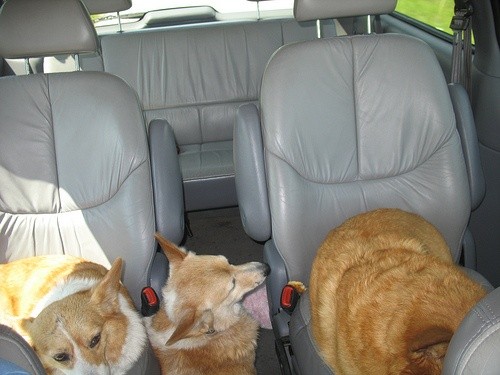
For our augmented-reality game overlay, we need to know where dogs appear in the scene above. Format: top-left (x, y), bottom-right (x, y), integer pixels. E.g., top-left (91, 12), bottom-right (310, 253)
top-left (308, 208), bottom-right (490, 375)
top-left (0, 254), bottom-right (149, 375)
top-left (140, 232), bottom-right (273, 375)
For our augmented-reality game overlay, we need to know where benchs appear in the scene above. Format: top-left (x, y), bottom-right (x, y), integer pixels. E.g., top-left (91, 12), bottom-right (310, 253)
top-left (8, 1), bottom-right (355, 212)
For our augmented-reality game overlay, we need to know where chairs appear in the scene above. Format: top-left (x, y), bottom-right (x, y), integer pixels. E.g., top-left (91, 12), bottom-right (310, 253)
top-left (0, 0), bottom-right (499, 375)
